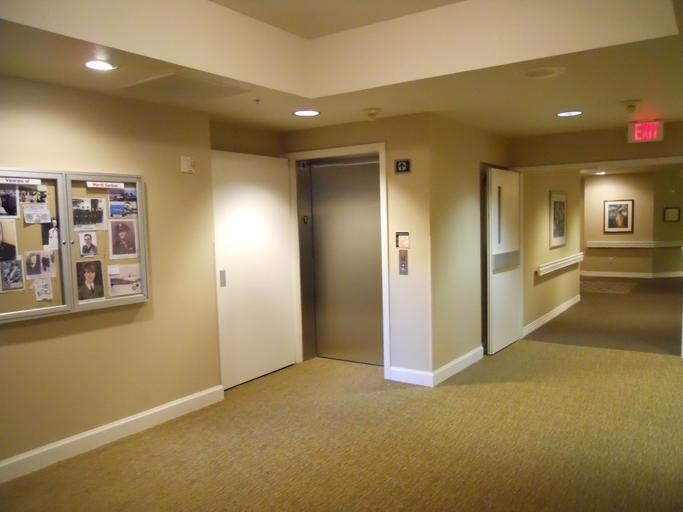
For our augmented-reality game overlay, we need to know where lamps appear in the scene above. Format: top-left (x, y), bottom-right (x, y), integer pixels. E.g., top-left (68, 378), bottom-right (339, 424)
top-left (363, 107), bottom-right (381, 119)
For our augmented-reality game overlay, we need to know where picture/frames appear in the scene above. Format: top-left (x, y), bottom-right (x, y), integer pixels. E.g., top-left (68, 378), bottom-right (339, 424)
top-left (549, 190), bottom-right (567, 249)
top-left (663, 207), bottom-right (680, 222)
top-left (603, 199), bottom-right (634, 233)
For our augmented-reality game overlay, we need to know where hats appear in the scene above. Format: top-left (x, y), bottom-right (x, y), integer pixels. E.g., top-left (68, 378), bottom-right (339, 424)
top-left (116, 222), bottom-right (129, 233)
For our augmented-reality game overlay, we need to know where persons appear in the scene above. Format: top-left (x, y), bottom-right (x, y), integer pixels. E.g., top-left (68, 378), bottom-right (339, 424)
top-left (81, 233), bottom-right (96, 254)
top-left (73, 204), bottom-right (103, 226)
top-left (26, 254), bottom-right (41, 277)
top-left (77, 261), bottom-right (102, 301)
top-left (17, 187), bottom-right (47, 204)
top-left (111, 221), bottom-right (135, 255)
top-left (5, 260), bottom-right (21, 286)
top-left (47, 219), bottom-right (59, 246)
